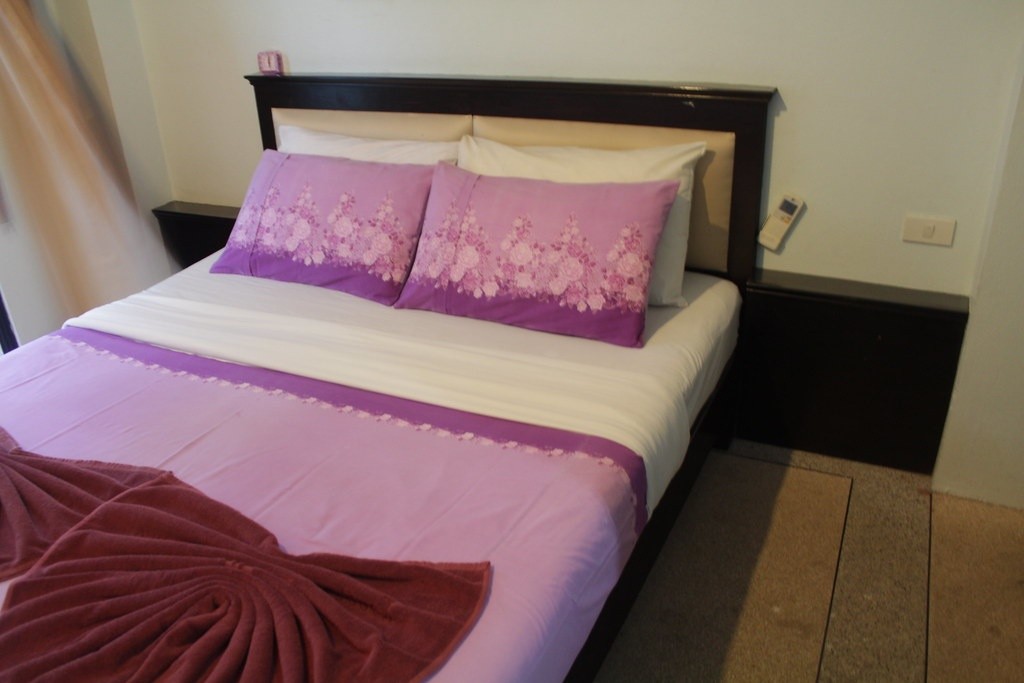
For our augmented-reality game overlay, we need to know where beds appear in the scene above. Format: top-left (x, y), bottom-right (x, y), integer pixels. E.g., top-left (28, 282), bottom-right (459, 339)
top-left (1, 74), bottom-right (778, 683)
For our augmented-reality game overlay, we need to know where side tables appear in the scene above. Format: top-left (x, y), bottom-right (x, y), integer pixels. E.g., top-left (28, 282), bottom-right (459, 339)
top-left (715, 268), bottom-right (970, 477)
top-left (150, 199), bottom-right (243, 267)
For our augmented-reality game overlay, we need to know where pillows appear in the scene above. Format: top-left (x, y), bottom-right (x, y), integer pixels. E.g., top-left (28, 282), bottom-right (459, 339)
top-left (211, 148), bottom-right (457, 308)
top-left (452, 135), bottom-right (707, 310)
top-left (394, 160), bottom-right (680, 351)
top-left (277, 122), bottom-right (457, 166)
